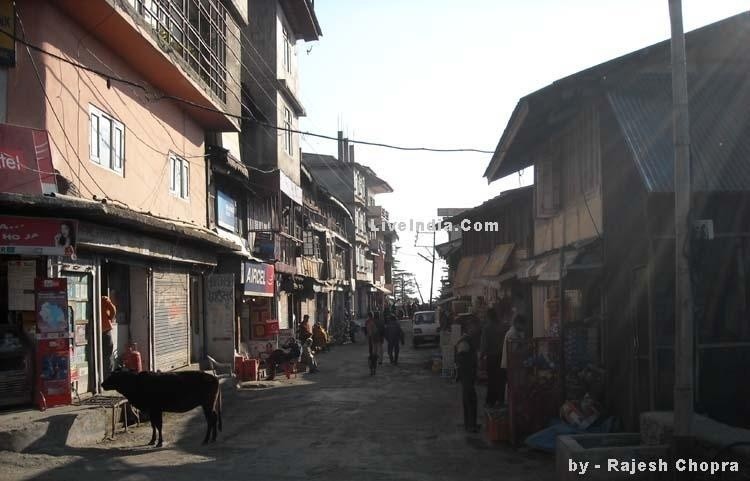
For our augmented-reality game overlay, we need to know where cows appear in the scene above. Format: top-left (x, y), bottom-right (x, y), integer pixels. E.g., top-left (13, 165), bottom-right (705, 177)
top-left (100, 364), bottom-right (223, 449)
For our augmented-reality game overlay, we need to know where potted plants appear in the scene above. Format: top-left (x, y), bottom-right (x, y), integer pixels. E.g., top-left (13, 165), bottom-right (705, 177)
top-left (487, 410), bottom-right (526, 441)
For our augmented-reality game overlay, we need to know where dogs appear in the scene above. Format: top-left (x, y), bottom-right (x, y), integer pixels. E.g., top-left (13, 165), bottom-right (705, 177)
top-left (368, 353), bottom-right (378, 375)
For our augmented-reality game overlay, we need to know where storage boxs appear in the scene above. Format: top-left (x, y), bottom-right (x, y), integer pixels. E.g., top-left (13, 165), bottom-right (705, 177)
top-left (429, 321), bottom-right (462, 377)
top-left (236, 357), bottom-right (272, 380)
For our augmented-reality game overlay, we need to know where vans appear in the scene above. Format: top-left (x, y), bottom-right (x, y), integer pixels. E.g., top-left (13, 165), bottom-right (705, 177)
top-left (411, 309), bottom-right (441, 344)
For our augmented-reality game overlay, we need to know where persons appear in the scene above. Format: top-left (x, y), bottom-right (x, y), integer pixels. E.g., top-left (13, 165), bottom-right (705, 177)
top-left (302, 315), bottom-right (311, 338)
top-left (367, 303), bottom-right (416, 378)
top-left (524, 358), bottom-right (606, 455)
top-left (453, 317), bottom-right (481, 434)
top-left (53, 222), bottom-right (75, 249)
top-left (478, 305), bottom-right (510, 410)
top-left (301, 338), bottom-right (320, 373)
top-left (257, 337), bottom-right (300, 380)
top-left (498, 313), bottom-right (527, 371)
